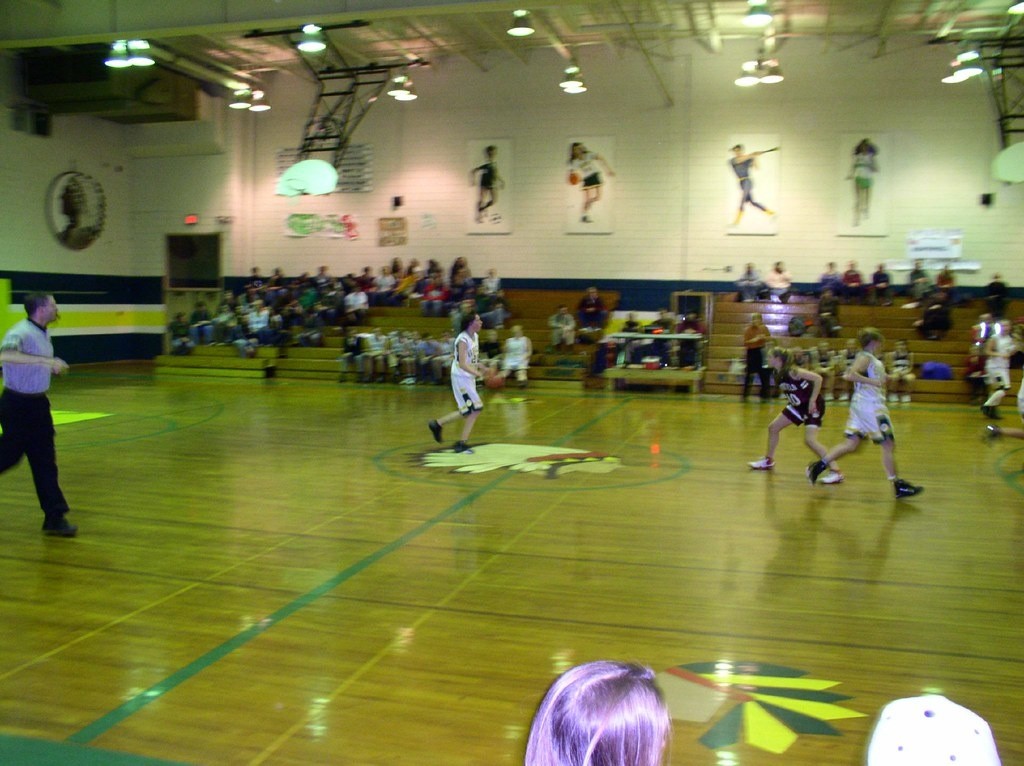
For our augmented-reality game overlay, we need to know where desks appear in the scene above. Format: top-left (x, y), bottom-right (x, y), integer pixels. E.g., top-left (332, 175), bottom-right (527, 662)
top-left (604, 330), bottom-right (706, 395)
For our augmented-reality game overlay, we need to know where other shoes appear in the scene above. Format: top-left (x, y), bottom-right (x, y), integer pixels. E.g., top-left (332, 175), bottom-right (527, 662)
top-left (454, 443), bottom-right (473, 454)
top-left (889, 393), bottom-right (899, 402)
top-left (901, 395), bottom-right (911, 403)
top-left (41, 515), bottom-right (78, 536)
top-left (428, 419), bottom-right (443, 445)
top-left (980, 405), bottom-right (1003, 418)
top-left (337, 371), bottom-right (451, 385)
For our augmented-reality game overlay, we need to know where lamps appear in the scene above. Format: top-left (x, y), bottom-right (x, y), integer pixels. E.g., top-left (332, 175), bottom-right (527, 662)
top-left (742, 0), bottom-right (771, 27)
top-left (734, 45), bottom-right (784, 86)
top-left (506, 9), bottom-right (536, 36)
top-left (559, 58), bottom-right (588, 94)
top-left (229, 88), bottom-right (272, 112)
top-left (298, 24), bottom-right (326, 53)
top-left (106, 39), bottom-right (155, 67)
top-left (387, 75), bottom-right (417, 101)
top-left (941, 41), bottom-right (983, 83)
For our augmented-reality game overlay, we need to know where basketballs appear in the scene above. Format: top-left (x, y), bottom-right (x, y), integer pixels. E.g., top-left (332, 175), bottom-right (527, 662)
top-left (484, 373), bottom-right (504, 389)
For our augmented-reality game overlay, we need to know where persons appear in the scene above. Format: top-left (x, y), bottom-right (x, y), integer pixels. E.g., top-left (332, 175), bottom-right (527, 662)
top-left (767, 341), bottom-right (916, 403)
top-left (819, 258), bottom-right (956, 308)
top-left (524, 660), bottom-right (674, 766)
top-left (729, 143), bottom-right (782, 226)
top-left (762, 261), bottom-right (793, 303)
top-left (738, 263), bottom-right (760, 303)
top-left (982, 272), bottom-right (1012, 320)
top-left (867, 694), bottom-right (1001, 766)
top-left (914, 298), bottom-right (954, 340)
top-left (428, 309), bottom-right (495, 452)
top-left (818, 286), bottom-right (842, 338)
top-left (959, 314), bottom-right (1024, 423)
top-left (0, 291), bottom-right (79, 537)
top-left (806, 327), bottom-right (924, 499)
top-left (612, 307), bottom-right (706, 370)
top-left (846, 138), bottom-right (879, 226)
top-left (339, 325), bottom-right (533, 389)
top-left (567, 142), bottom-right (615, 224)
top-left (470, 145), bottom-right (505, 224)
top-left (577, 287), bottom-right (608, 332)
top-left (166, 256), bottom-right (512, 360)
top-left (547, 302), bottom-right (577, 355)
top-left (739, 314), bottom-right (773, 403)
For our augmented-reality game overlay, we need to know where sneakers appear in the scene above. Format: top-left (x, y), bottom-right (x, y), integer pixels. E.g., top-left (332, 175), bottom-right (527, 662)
top-left (805, 460), bottom-right (827, 485)
top-left (821, 472), bottom-right (845, 484)
top-left (747, 458), bottom-right (775, 470)
top-left (893, 479), bottom-right (922, 498)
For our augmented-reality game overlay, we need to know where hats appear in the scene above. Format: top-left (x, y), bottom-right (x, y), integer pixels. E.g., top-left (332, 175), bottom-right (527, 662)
top-left (868, 694), bottom-right (1001, 766)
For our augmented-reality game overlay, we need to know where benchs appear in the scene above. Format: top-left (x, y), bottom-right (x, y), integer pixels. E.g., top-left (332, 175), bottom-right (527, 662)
top-left (153, 290), bottom-right (621, 380)
top-left (702, 290), bottom-right (1024, 406)
